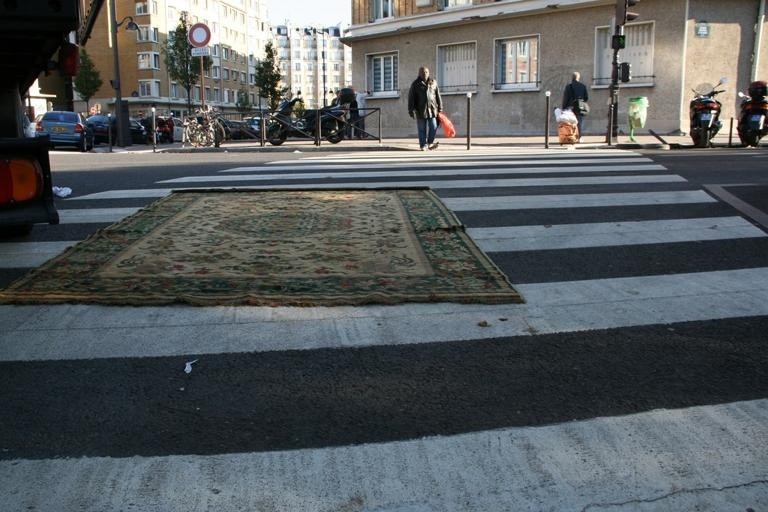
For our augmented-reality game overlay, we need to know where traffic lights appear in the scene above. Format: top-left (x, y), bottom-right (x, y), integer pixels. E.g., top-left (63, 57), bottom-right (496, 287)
top-left (611, 34), bottom-right (626, 50)
top-left (616, 1), bottom-right (639, 28)
top-left (622, 61), bottom-right (632, 84)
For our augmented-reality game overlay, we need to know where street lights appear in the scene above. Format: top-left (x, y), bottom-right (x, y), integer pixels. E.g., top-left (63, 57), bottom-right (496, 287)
top-left (107, 15), bottom-right (139, 146)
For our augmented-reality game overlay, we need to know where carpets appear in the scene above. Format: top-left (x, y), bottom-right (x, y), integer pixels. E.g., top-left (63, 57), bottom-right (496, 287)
top-left (1, 184), bottom-right (528, 307)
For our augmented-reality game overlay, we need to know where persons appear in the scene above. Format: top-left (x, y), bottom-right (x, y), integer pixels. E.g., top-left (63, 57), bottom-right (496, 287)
top-left (408, 67), bottom-right (442, 151)
top-left (347, 85), bottom-right (371, 135)
top-left (562, 72), bottom-right (588, 140)
top-left (331, 91), bottom-right (344, 136)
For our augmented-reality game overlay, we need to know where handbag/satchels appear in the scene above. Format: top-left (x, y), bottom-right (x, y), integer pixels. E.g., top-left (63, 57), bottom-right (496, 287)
top-left (572, 97), bottom-right (590, 117)
top-left (437, 110), bottom-right (456, 138)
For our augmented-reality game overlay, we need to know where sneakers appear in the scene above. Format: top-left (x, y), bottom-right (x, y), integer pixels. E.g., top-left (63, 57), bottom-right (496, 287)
top-left (420, 141), bottom-right (439, 152)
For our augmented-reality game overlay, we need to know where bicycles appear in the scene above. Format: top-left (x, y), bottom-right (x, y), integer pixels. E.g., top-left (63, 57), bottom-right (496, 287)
top-left (182, 105), bottom-right (227, 149)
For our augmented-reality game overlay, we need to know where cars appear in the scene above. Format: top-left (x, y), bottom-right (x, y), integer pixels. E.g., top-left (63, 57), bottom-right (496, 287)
top-left (27, 111), bottom-right (182, 152)
top-left (225, 114), bottom-right (306, 139)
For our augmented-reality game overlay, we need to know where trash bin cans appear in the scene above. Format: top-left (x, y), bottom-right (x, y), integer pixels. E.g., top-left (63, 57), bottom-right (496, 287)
top-left (628, 97), bottom-right (649, 143)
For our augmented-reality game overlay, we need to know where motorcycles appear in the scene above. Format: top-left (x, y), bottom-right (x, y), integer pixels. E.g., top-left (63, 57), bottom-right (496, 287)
top-left (688, 76), bottom-right (729, 148)
top-left (735, 80), bottom-right (767, 149)
top-left (266, 88), bottom-right (347, 145)
